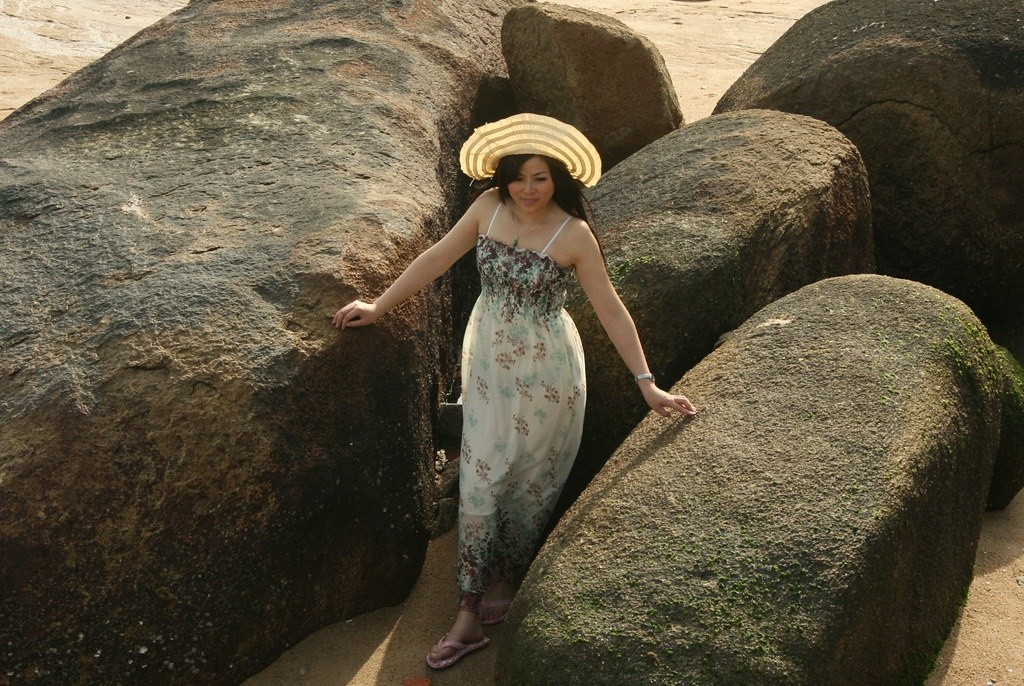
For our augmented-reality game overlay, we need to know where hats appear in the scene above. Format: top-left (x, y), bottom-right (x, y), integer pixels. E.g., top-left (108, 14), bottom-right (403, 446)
top-left (459, 113), bottom-right (601, 187)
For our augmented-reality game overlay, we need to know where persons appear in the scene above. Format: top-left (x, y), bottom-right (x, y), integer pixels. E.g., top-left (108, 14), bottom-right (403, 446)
top-left (332, 113), bottom-right (697, 668)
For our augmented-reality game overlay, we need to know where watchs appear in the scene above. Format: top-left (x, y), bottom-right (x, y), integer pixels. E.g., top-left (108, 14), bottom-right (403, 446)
top-left (635, 374), bottom-right (656, 383)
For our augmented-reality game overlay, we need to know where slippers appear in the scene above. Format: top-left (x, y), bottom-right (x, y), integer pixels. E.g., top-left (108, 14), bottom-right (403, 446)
top-left (478, 598), bottom-right (513, 625)
top-left (426, 632), bottom-right (490, 668)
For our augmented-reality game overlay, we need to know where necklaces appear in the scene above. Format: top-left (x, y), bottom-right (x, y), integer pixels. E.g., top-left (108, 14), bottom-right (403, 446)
top-left (512, 201), bottom-right (553, 248)
